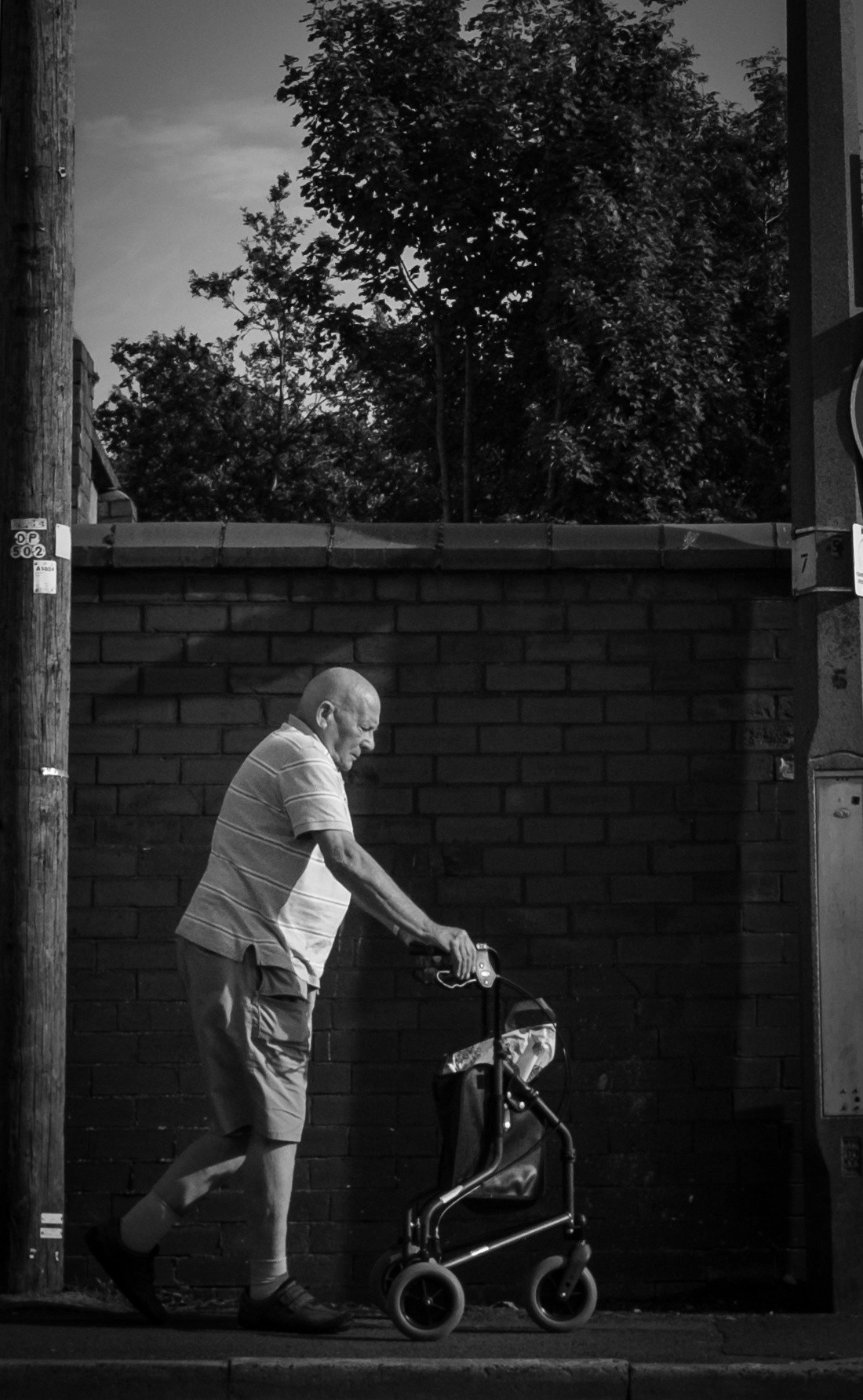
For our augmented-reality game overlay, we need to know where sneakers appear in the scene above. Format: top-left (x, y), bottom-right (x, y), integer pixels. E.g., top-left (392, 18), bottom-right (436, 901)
top-left (236, 1278), bottom-right (354, 1335)
top-left (91, 1228), bottom-right (171, 1329)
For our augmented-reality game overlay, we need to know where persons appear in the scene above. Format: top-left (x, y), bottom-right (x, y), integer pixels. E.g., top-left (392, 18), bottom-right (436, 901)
top-left (94, 668), bottom-right (477, 1338)
